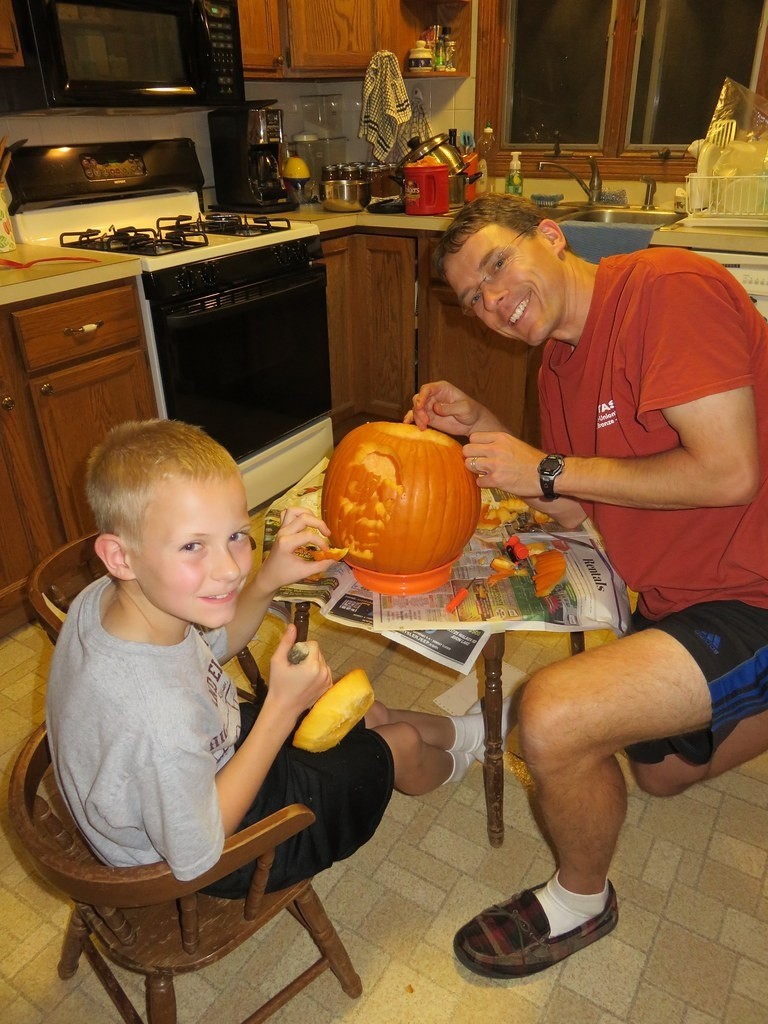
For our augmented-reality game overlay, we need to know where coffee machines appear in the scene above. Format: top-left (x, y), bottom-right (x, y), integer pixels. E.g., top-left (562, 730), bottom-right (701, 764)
top-left (205, 99), bottom-right (299, 211)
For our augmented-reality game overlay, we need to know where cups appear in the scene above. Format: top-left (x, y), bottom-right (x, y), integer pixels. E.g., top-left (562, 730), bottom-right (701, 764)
top-left (408, 39), bottom-right (434, 72)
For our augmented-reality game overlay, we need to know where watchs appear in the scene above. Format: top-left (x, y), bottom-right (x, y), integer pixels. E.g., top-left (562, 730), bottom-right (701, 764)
top-left (537, 454), bottom-right (566, 499)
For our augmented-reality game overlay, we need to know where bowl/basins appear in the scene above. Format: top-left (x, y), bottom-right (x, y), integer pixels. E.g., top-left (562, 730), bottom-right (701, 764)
top-left (713, 141), bottom-right (758, 178)
top-left (319, 179), bottom-right (369, 213)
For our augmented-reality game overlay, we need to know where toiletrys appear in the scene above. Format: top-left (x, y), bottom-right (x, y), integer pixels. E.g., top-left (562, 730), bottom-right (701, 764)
top-left (477, 119), bottom-right (496, 193)
top-left (504, 152), bottom-right (523, 197)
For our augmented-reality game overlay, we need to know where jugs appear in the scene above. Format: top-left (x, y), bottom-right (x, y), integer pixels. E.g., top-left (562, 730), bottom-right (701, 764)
top-left (403, 164), bottom-right (450, 216)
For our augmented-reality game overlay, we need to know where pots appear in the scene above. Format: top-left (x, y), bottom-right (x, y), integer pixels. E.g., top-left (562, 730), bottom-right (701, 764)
top-left (400, 131), bottom-right (484, 215)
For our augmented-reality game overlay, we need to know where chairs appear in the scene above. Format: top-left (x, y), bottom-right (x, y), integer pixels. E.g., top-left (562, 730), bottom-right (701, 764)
top-left (28, 532), bottom-right (269, 705)
top-left (8, 720), bottom-right (363, 1024)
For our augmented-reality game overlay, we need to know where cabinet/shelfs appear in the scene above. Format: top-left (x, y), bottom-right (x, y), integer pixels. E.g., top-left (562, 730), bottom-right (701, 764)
top-left (0, 276), bottom-right (160, 640)
top-left (236, 0), bottom-right (473, 84)
top-left (0, 0), bottom-right (24, 67)
top-left (317, 234), bottom-right (542, 449)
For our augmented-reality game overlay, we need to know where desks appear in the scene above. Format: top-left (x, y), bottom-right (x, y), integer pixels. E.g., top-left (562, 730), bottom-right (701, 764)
top-left (293, 601), bottom-right (586, 847)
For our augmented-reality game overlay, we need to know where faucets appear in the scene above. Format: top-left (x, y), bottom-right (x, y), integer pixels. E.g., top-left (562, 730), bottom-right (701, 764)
top-left (537, 155), bottom-right (602, 202)
top-left (639, 175), bottom-right (657, 210)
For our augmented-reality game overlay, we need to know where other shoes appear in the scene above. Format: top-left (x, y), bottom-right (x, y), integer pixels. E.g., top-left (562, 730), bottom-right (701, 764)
top-left (453, 877), bottom-right (619, 978)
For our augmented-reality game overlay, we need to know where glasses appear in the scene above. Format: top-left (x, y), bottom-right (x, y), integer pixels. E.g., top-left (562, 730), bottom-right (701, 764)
top-left (459, 220), bottom-right (543, 318)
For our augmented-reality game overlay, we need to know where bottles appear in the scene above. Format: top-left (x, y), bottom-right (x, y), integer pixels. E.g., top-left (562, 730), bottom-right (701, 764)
top-left (447, 128), bottom-right (461, 152)
top-left (434, 27), bottom-right (457, 72)
top-left (324, 160), bottom-right (403, 198)
top-left (473, 121), bottom-right (499, 198)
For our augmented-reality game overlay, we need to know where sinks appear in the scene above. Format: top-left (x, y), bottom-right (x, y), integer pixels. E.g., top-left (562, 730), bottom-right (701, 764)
top-left (542, 207), bottom-right (579, 220)
top-left (561, 211), bottom-right (688, 231)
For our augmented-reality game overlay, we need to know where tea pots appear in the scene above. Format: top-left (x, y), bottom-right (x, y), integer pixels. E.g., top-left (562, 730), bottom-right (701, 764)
top-left (246, 149), bottom-right (280, 193)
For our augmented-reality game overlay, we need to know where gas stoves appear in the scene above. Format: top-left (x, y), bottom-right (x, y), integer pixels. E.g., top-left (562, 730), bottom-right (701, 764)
top-left (3, 136), bottom-right (319, 272)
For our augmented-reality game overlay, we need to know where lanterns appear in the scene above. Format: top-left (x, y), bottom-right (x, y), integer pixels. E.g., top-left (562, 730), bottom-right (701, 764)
top-left (320, 420), bottom-right (482, 575)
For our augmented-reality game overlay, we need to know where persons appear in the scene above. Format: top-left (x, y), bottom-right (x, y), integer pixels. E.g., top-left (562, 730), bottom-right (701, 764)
top-left (401, 193), bottom-right (768, 977)
top-left (44, 417), bottom-right (529, 902)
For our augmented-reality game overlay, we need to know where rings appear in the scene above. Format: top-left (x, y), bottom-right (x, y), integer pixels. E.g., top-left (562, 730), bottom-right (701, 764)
top-left (471, 457), bottom-right (477, 472)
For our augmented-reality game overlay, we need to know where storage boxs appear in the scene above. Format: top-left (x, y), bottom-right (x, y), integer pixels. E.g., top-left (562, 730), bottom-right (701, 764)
top-left (301, 94), bottom-right (347, 188)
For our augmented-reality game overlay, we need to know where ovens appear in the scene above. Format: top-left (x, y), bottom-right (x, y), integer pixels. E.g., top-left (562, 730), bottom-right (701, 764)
top-left (0, 0), bottom-right (245, 113)
top-left (135, 234), bottom-right (334, 520)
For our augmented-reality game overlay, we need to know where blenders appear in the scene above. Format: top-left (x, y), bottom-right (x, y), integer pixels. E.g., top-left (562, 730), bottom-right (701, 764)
top-left (281, 130), bottom-right (318, 207)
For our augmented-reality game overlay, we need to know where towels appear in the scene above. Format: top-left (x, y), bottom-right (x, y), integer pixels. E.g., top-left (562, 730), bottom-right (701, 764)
top-left (410, 99), bottom-right (433, 143)
top-left (558, 221), bottom-right (665, 264)
top-left (357, 50), bottom-right (412, 163)
